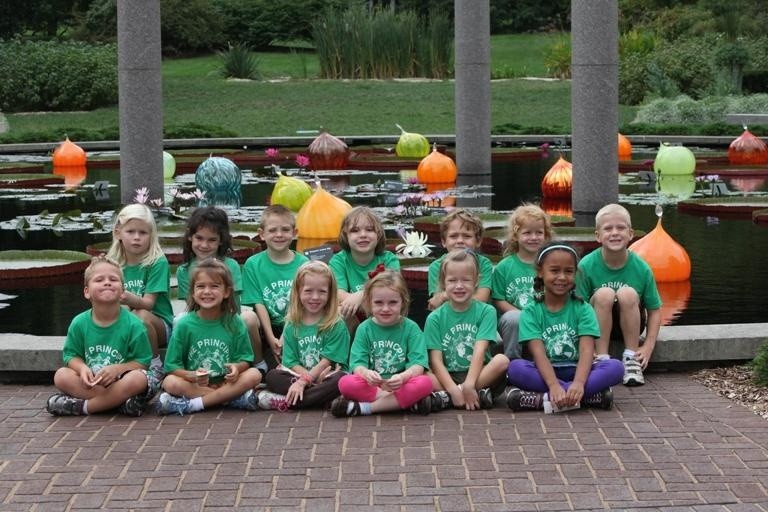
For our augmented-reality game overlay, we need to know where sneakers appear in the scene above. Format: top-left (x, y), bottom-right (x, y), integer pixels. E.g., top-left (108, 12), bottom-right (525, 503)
top-left (431, 390), bottom-right (451, 410)
top-left (158, 392), bottom-right (192, 416)
top-left (231, 387), bottom-right (258, 412)
top-left (119, 395), bottom-right (145, 418)
top-left (620, 349), bottom-right (646, 388)
top-left (476, 387), bottom-right (495, 408)
top-left (45, 393), bottom-right (87, 417)
top-left (409, 394), bottom-right (436, 415)
top-left (330, 396), bottom-right (363, 418)
top-left (587, 385), bottom-right (615, 411)
top-left (506, 387), bottom-right (549, 413)
top-left (255, 389), bottom-right (290, 413)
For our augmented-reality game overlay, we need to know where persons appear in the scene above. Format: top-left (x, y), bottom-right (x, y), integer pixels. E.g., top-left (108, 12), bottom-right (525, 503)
top-left (45, 254), bottom-right (152, 417)
top-left (155, 259), bottom-right (262, 416)
top-left (424, 248), bottom-right (510, 410)
top-left (575, 204), bottom-right (663, 387)
top-left (239, 204), bottom-right (311, 382)
top-left (176, 206), bottom-right (242, 315)
top-left (328, 206), bottom-right (402, 340)
top-left (330, 270), bottom-right (434, 415)
top-left (253, 259), bottom-right (351, 411)
top-left (104, 203), bottom-right (176, 369)
top-left (427, 210), bottom-right (494, 310)
top-left (507, 244), bottom-right (625, 412)
top-left (491, 204), bottom-right (555, 360)
